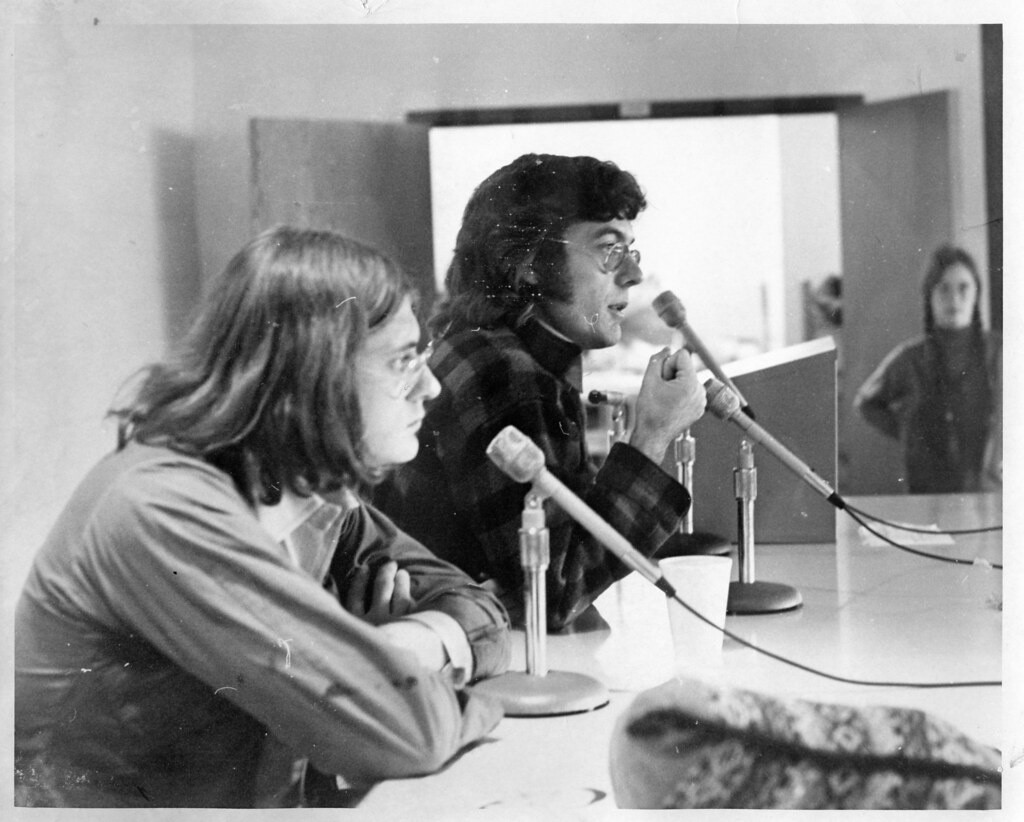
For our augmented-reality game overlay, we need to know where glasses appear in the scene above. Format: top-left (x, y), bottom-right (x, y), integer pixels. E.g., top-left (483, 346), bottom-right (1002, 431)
top-left (362, 341), bottom-right (434, 402)
top-left (543, 234), bottom-right (641, 274)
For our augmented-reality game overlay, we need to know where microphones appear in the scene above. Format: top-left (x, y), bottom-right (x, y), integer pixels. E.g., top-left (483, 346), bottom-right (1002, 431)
top-left (703, 377), bottom-right (844, 509)
top-left (652, 290), bottom-right (756, 422)
top-left (485, 423), bottom-right (676, 601)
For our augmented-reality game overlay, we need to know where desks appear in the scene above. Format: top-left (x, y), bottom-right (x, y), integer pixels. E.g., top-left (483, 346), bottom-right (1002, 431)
top-left (358, 493), bottom-right (1002, 809)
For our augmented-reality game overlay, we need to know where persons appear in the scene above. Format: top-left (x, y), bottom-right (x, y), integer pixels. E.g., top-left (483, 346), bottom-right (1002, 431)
top-left (14, 224), bottom-right (509, 809)
top-left (852, 243), bottom-right (1003, 493)
top-left (368, 154), bottom-right (708, 635)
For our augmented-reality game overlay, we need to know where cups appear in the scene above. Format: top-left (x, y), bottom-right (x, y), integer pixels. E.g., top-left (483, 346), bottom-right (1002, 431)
top-left (658, 555), bottom-right (733, 660)
